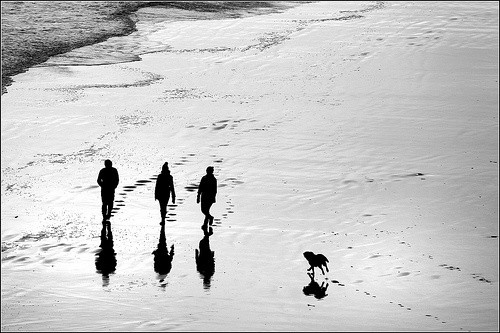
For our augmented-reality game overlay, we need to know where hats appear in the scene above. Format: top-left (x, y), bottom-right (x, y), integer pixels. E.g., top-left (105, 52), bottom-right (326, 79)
top-left (162, 162), bottom-right (168, 169)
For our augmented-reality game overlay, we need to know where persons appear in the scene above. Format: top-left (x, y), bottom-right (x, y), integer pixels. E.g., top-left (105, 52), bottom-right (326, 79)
top-left (196, 166), bottom-right (217, 229)
top-left (155, 162), bottom-right (176, 226)
top-left (97, 159), bottom-right (119, 221)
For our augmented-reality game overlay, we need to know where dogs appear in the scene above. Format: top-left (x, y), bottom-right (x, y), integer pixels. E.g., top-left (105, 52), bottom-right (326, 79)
top-left (303, 251), bottom-right (330, 276)
top-left (302, 273), bottom-right (329, 299)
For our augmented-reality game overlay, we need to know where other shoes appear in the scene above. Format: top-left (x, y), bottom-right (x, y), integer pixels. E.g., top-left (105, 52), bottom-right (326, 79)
top-left (102, 220), bottom-right (106, 224)
top-left (203, 229), bottom-right (208, 234)
top-left (103, 224), bottom-right (106, 227)
top-left (107, 214), bottom-right (111, 219)
top-left (161, 224), bottom-right (164, 226)
top-left (202, 225), bottom-right (207, 229)
top-left (160, 221), bottom-right (165, 224)
top-left (106, 221), bottom-right (111, 226)
top-left (209, 216), bottom-right (214, 225)
top-left (209, 227), bottom-right (213, 235)
